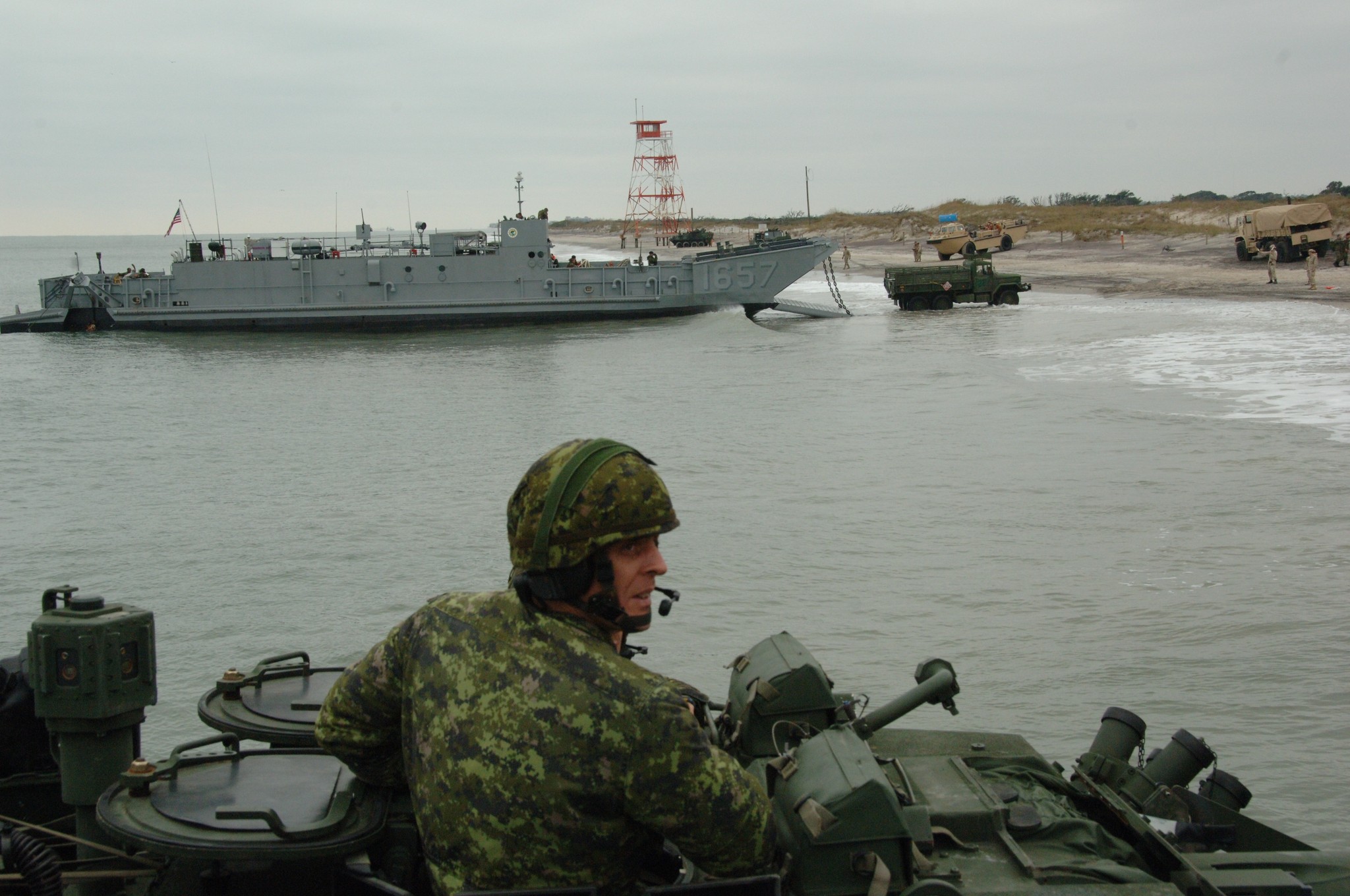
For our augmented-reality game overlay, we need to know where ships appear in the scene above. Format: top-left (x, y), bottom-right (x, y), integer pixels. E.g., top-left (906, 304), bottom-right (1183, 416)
top-left (0, 172), bottom-right (854, 334)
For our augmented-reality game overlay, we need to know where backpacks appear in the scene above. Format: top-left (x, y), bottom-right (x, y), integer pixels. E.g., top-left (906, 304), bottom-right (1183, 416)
top-left (1330, 240), bottom-right (1335, 251)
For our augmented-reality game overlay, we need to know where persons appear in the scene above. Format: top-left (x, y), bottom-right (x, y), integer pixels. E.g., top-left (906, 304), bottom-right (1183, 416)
top-left (912, 242), bottom-right (922, 262)
top-left (538, 208), bottom-right (582, 268)
top-left (647, 251), bottom-right (658, 265)
top-left (312, 438), bottom-right (782, 896)
top-left (985, 222), bottom-right (1002, 234)
top-left (841, 246), bottom-right (851, 270)
top-left (1257, 233), bottom-right (1350, 290)
top-left (117, 264), bottom-right (149, 278)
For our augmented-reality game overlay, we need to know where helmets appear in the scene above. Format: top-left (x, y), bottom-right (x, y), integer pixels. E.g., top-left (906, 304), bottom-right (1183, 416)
top-left (507, 440), bottom-right (680, 571)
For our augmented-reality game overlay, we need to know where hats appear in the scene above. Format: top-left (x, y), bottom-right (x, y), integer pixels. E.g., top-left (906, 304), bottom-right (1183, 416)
top-left (1269, 245), bottom-right (1276, 248)
top-left (1307, 249), bottom-right (1315, 252)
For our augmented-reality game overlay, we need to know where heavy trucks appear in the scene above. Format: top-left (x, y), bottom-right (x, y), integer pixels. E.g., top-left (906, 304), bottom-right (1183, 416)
top-left (1234, 203), bottom-right (1332, 264)
top-left (884, 252), bottom-right (1031, 313)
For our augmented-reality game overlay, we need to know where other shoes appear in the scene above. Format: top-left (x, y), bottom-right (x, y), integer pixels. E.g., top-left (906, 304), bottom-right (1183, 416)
top-left (1266, 280), bottom-right (1273, 284)
top-left (1337, 263), bottom-right (1341, 267)
top-left (1273, 279), bottom-right (1277, 283)
top-left (1333, 262), bottom-right (1338, 268)
top-left (1344, 263), bottom-right (1349, 266)
top-left (1308, 285), bottom-right (1312, 289)
top-left (1310, 284), bottom-right (1317, 290)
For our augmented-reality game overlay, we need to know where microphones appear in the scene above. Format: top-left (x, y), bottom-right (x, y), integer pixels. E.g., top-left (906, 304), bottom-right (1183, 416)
top-left (653, 586), bottom-right (681, 616)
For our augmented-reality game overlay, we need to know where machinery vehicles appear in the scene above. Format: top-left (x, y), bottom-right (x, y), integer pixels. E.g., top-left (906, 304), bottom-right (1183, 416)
top-left (669, 228), bottom-right (714, 248)
top-left (753, 227), bottom-right (792, 244)
top-left (926, 219), bottom-right (1028, 261)
